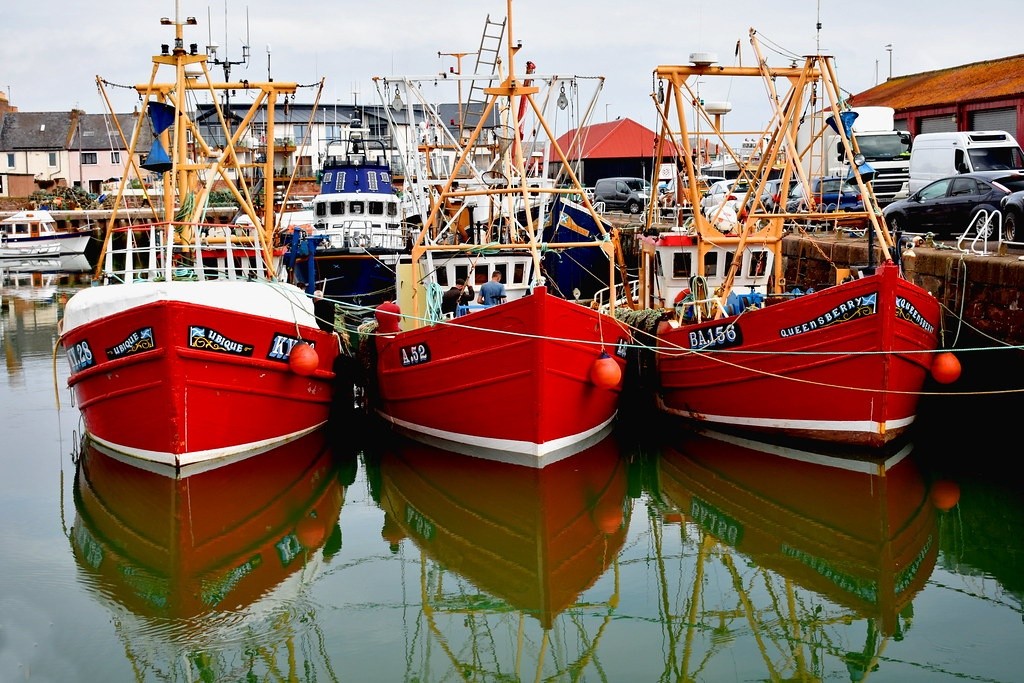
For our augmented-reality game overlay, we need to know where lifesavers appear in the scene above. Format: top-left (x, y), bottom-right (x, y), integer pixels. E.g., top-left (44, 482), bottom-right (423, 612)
top-left (673, 289), bottom-right (695, 321)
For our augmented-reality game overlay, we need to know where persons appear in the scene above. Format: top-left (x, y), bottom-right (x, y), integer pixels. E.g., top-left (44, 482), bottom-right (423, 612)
top-left (141, 186), bottom-right (151, 208)
top-left (477, 271), bottom-right (507, 309)
top-left (313, 290), bottom-right (323, 302)
top-left (375, 292), bottom-right (403, 335)
top-left (442, 279), bottom-right (475, 318)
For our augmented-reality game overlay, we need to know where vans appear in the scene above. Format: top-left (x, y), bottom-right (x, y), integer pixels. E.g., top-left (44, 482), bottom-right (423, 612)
top-left (658, 173), bottom-right (728, 221)
top-left (592, 176), bottom-right (661, 214)
top-left (909, 130), bottom-right (1024, 199)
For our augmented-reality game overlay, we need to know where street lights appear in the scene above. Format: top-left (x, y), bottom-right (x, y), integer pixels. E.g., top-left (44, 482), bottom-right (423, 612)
top-left (884, 44), bottom-right (893, 79)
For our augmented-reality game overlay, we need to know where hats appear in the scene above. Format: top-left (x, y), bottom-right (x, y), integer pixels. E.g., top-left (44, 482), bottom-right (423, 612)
top-left (456, 279), bottom-right (465, 285)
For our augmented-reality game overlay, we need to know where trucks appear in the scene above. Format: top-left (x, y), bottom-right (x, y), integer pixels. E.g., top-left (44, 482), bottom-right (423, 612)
top-left (797, 103), bottom-right (915, 209)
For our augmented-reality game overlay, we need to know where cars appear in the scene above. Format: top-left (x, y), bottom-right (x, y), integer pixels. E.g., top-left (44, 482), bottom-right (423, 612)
top-left (1001, 189), bottom-right (1024, 250)
top-left (699, 178), bottom-right (759, 217)
top-left (745, 178), bottom-right (800, 217)
top-left (879, 169), bottom-right (1024, 242)
top-left (785, 173), bottom-right (868, 232)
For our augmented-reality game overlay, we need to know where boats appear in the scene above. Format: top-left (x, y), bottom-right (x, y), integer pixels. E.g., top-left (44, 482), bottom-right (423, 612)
top-left (52, 0), bottom-right (345, 469)
top-left (204, 1), bottom-right (616, 312)
top-left (60, 416), bottom-right (353, 683)
top-left (641, 398), bottom-right (941, 683)
top-left (631, 0), bottom-right (945, 477)
top-left (0, 208), bottom-right (101, 257)
top-left (354, 0), bottom-right (638, 472)
top-left (356, 402), bottom-right (637, 683)
